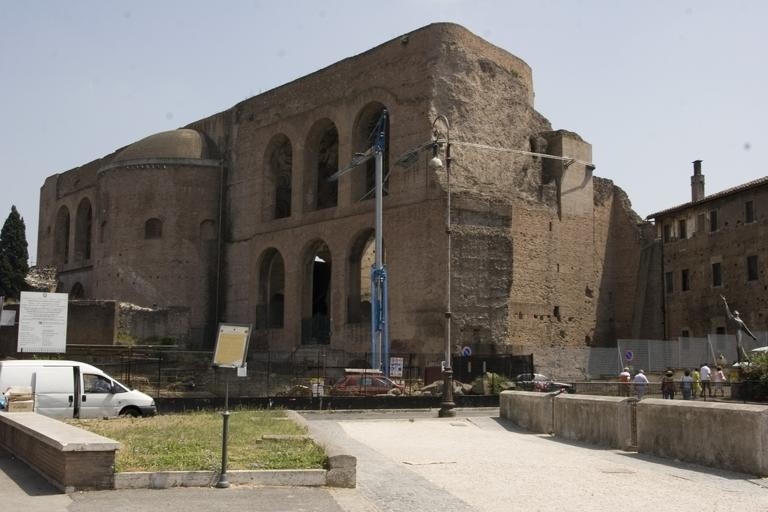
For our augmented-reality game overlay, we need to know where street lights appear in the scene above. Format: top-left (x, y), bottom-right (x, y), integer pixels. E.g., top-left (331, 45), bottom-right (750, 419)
top-left (429, 114), bottom-right (453, 402)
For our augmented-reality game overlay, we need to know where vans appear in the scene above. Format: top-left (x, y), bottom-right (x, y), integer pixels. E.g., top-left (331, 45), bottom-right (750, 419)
top-left (0, 359), bottom-right (157, 418)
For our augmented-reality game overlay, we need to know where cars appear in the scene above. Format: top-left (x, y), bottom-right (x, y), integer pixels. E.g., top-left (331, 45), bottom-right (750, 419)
top-left (329, 374), bottom-right (404, 396)
top-left (513, 373), bottom-right (575, 392)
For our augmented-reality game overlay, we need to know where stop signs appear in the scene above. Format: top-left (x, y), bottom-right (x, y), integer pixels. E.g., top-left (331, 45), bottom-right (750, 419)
top-left (462, 347), bottom-right (472, 357)
top-left (625, 350), bottom-right (633, 361)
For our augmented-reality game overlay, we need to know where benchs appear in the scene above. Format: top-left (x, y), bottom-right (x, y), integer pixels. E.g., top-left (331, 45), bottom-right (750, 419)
top-left (0, 408), bottom-right (120, 494)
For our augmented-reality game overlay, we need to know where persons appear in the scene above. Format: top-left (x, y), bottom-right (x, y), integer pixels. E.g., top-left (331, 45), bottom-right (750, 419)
top-left (633, 369), bottom-right (649, 401)
top-left (700, 363), bottom-right (713, 395)
top-left (679, 369), bottom-right (693, 400)
top-left (617, 367), bottom-right (631, 397)
top-left (690, 368), bottom-right (703, 398)
top-left (719, 294), bottom-right (758, 362)
top-left (713, 366), bottom-right (727, 397)
top-left (661, 370), bottom-right (676, 398)
